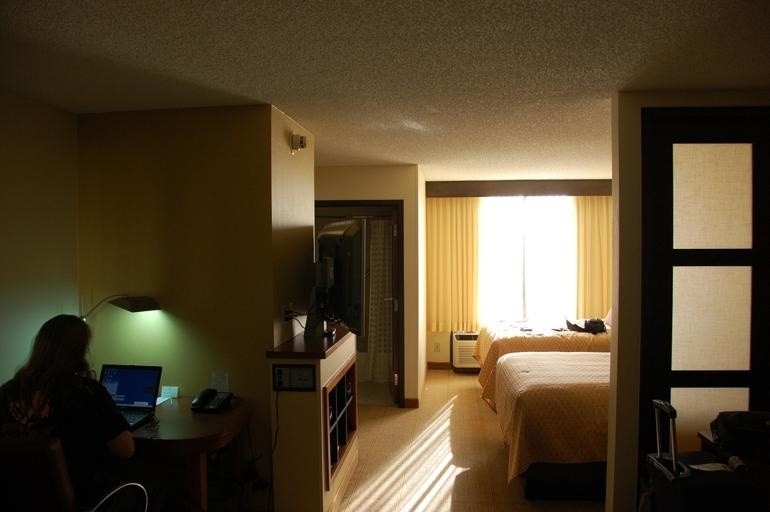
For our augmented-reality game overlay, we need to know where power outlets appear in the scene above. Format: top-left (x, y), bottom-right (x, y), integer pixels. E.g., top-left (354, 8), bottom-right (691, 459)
top-left (272, 364), bottom-right (316, 392)
top-left (280, 302), bottom-right (295, 324)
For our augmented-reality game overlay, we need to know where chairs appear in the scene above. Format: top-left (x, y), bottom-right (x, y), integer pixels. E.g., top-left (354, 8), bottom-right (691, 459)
top-left (0, 432), bottom-right (148, 511)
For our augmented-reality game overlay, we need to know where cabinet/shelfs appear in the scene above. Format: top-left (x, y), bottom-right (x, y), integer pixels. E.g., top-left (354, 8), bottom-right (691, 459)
top-left (272, 334), bottom-right (358, 511)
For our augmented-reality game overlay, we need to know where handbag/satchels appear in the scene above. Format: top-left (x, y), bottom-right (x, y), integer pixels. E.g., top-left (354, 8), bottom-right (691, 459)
top-left (241, 478), bottom-right (271, 512)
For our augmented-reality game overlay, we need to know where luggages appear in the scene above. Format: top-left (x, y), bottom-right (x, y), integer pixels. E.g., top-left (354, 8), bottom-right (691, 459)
top-left (642, 396), bottom-right (692, 512)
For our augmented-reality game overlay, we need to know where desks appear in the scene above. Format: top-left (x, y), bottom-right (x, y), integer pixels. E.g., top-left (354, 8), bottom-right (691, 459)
top-left (697, 431), bottom-right (770, 512)
top-left (132, 398), bottom-right (256, 512)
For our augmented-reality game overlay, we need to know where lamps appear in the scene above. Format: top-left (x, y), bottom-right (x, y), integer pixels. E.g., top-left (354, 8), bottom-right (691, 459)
top-left (79, 294), bottom-right (159, 321)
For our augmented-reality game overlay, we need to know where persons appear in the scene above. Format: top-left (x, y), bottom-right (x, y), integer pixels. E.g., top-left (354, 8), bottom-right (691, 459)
top-left (1, 314), bottom-right (149, 511)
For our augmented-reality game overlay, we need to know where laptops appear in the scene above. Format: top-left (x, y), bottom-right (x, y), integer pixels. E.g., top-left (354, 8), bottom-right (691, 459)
top-left (99, 364), bottom-right (162, 431)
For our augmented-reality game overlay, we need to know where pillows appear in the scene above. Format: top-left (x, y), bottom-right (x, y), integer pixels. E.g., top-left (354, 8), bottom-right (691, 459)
top-left (605, 309), bottom-right (612, 327)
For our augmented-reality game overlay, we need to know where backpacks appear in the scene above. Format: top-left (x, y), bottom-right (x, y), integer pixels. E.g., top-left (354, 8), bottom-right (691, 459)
top-left (708, 409), bottom-right (770, 455)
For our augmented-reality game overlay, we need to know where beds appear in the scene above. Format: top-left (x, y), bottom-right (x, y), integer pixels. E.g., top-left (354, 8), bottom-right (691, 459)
top-left (478, 352), bottom-right (610, 503)
top-left (473, 316), bottom-right (613, 417)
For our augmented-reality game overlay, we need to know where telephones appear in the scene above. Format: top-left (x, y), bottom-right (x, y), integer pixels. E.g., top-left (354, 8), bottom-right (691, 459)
top-left (191, 389), bottom-right (233, 411)
